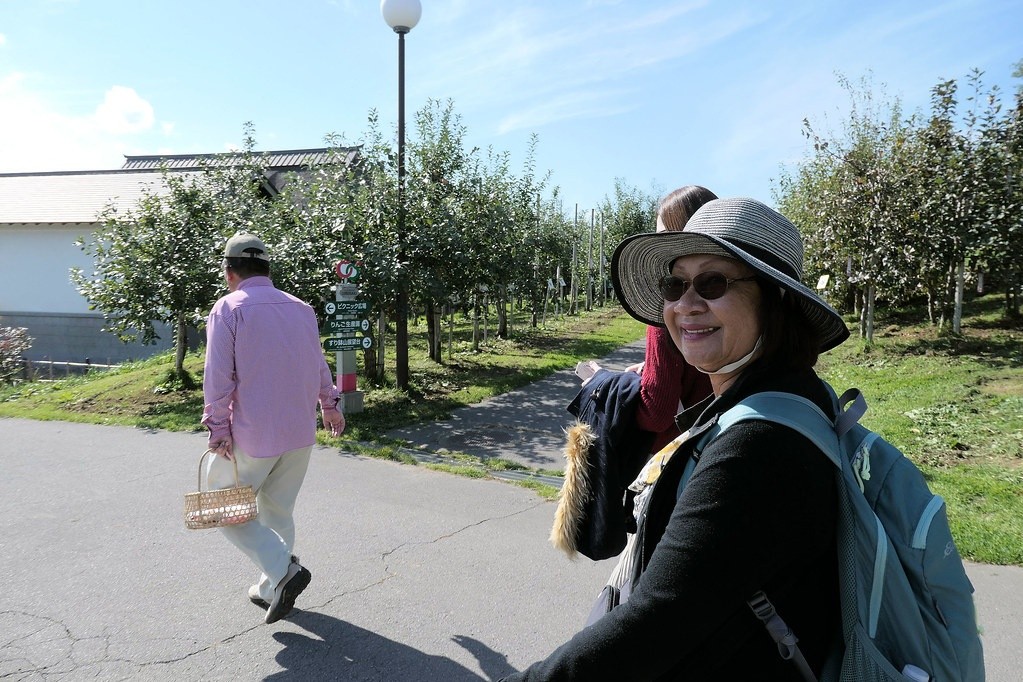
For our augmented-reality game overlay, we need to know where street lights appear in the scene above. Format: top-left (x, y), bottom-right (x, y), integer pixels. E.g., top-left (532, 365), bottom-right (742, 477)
top-left (383, 0), bottom-right (421, 396)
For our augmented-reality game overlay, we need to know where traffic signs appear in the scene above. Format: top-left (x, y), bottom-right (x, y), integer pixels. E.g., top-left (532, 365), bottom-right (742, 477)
top-left (325, 320), bottom-right (372, 334)
top-left (322, 337), bottom-right (375, 351)
top-left (323, 300), bottom-right (371, 317)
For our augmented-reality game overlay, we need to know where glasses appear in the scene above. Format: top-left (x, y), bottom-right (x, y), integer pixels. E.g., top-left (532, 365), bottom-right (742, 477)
top-left (658, 270), bottom-right (757, 302)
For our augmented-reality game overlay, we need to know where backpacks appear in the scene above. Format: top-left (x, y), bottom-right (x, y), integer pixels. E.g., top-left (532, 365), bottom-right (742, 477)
top-left (677, 379), bottom-right (987, 682)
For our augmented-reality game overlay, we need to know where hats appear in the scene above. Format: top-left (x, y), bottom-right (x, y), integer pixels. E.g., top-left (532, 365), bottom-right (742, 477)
top-left (610, 196), bottom-right (850, 354)
top-left (224, 234), bottom-right (269, 263)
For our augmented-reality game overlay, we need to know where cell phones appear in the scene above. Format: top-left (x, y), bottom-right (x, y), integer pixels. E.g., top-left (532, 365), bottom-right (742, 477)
top-left (575, 361), bottom-right (594, 381)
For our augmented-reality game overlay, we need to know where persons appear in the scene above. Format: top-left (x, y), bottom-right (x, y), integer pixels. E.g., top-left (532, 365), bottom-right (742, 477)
top-left (497, 197), bottom-right (851, 682)
top-left (581, 185), bottom-right (719, 461)
top-left (203, 233), bottom-right (346, 623)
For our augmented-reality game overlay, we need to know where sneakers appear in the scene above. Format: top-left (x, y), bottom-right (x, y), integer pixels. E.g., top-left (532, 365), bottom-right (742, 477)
top-left (264, 562), bottom-right (311, 624)
top-left (249, 585), bottom-right (264, 603)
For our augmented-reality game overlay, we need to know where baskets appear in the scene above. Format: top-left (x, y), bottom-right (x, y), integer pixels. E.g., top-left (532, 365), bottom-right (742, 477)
top-left (184, 447), bottom-right (257, 530)
